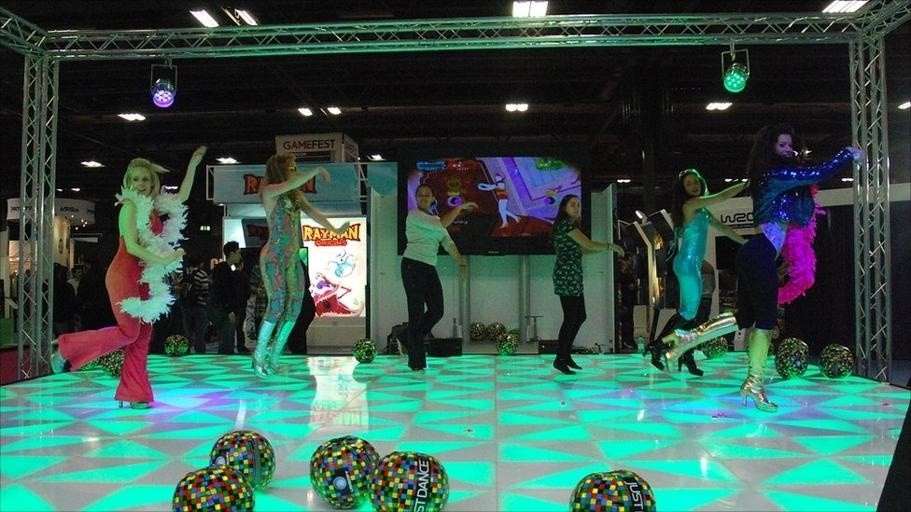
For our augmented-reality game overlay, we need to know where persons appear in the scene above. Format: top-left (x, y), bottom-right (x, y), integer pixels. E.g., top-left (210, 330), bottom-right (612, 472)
top-left (235, 258), bottom-right (316, 354)
top-left (662, 122), bottom-right (866, 412)
top-left (689, 259), bottom-right (715, 328)
top-left (176, 252), bottom-right (209, 354)
top-left (552, 194), bottom-right (625, 376)
top-left (642, 168), bottom-right (752, 376)
top-left (621, 254), bottom-right (638, 349)
top-left (477, 172), bottom-right (521, 229)
top-left (391, 184), bottom-right (480, 372)
top-left (15, 268), bottom-right (27, 321)
top-left (618, 259), bottom-right (628, 343)
top-left (50, 145), bottom-right (207, 408)
top-left (252, 153), bottom-right (348, 378)
top-left (38, 261), bottom-right (116, 327)
top-left (210, 241), bottom-right (241, 354)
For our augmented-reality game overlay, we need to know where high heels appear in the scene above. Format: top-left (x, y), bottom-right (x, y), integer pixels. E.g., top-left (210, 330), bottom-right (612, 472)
top-left (51, 335), bottom-right (68, 378)
top-left (406, 354), bottom-right (429, 371)
top-left (116, 395), bottom-right (151, 412)
top-left (391, 324), bottom-right (409, 356)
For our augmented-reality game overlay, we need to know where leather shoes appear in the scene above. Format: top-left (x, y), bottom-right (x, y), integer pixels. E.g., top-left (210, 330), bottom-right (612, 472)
top-left (554, 358), bottom-right (574, 375)
top-left (568, 355), bottom-right (581, 369)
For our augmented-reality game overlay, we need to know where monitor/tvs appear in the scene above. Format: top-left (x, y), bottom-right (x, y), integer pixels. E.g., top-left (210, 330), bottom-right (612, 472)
top-left (397, 145), bottom-right (594, 257)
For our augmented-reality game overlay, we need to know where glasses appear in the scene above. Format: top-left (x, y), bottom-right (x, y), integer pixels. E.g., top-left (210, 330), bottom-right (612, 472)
top-left (283, 163), bottom-right (299, 173)
top-left (679, 168), bottom-right (697, 182)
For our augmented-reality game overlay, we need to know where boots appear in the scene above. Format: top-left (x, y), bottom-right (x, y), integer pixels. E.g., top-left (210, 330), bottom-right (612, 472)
top-left (250, 318), bottom-right (295, 379)
top-left (664, 310), bottom-right (740, 374)
top-left (741, 329), bottom-right (778, 413)
top-left (642, 312), bottom-right (711, 376)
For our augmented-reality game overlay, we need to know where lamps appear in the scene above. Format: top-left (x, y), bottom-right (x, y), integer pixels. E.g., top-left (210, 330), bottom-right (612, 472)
top-left (146, 55), bottom-right (176, 110)
top-left (720, 38), bottom-right (751, 94)
